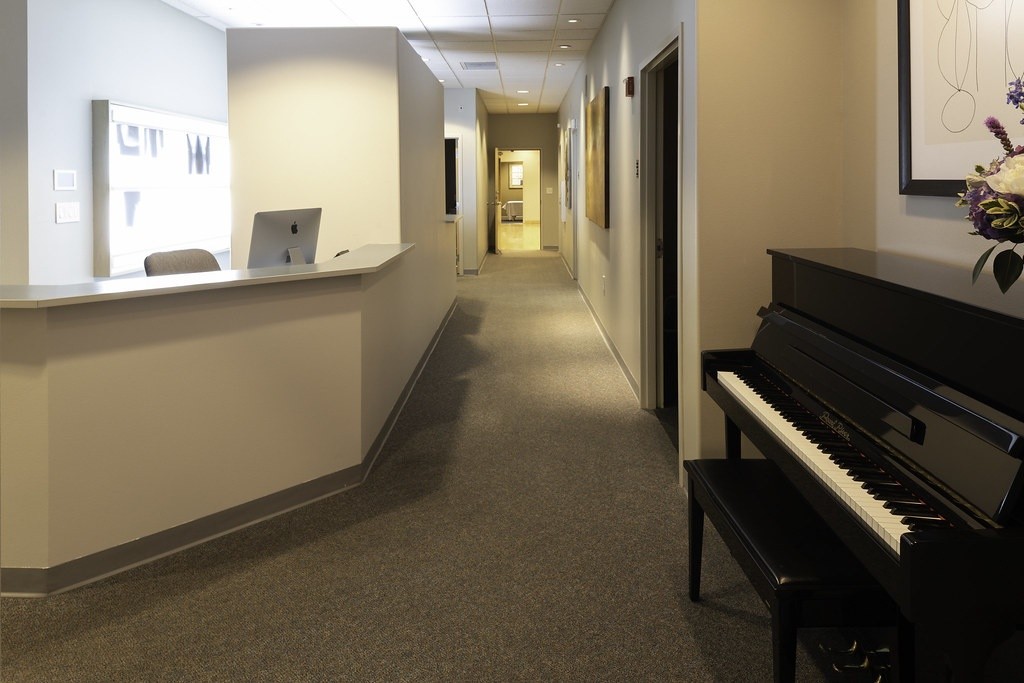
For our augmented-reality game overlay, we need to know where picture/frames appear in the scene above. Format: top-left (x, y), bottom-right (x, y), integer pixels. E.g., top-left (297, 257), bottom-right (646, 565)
top-left (896, 0), bottom-right (1024, 196)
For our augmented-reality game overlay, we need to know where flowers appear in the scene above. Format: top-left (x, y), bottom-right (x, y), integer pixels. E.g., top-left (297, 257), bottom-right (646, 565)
top-left (952, 76), bottom-right (1024, 295)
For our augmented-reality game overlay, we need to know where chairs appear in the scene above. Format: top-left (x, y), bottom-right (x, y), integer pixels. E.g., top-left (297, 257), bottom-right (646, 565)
top-left (144, 249), bottom-right (222, 278)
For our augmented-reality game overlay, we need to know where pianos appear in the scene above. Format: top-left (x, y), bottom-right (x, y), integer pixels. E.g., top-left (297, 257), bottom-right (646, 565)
top-left (698, 242), bottom-right (1023, 683)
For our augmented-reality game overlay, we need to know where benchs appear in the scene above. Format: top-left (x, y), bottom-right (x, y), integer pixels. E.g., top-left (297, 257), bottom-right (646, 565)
top-left (683, 457), bottom-right (916, 683)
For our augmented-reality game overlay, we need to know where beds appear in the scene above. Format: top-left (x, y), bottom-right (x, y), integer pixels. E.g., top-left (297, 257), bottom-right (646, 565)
top-left (501, 201), bottom-right (523, 221)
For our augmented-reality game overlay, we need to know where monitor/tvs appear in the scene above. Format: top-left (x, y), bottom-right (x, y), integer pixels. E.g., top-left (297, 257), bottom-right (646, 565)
top-left (247, 207), bottom-right (323, 269)
